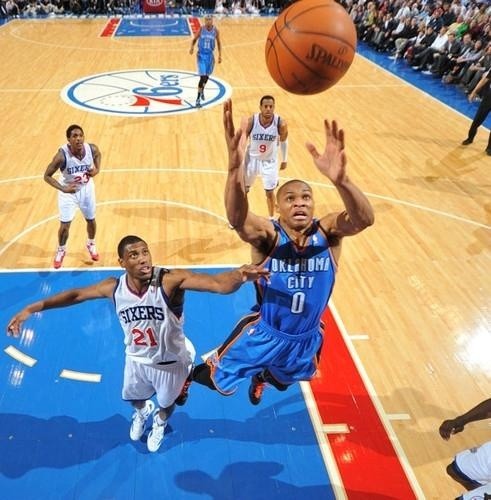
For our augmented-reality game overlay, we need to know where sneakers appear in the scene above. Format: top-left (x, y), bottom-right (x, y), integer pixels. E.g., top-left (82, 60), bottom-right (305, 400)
top-left (202, 91), bottom-right (206, 102)
top-left (195, 98), bottom-right (201, 108)
top-left (175, 380), bottom-right (192, 406)
top-left (53, 248), bottom-right (66, 269)
top-left (147, 408), bottom-right (168, 452)
top-left (128, 401), bottom-right (154, 440)
top-left (85, 241), bottom-right (100, 261)
top-left (249, 374), bottom-right (266, 404)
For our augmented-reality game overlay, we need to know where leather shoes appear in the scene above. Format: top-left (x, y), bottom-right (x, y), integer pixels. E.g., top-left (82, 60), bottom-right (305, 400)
top-left (462, 138), bottom-right (474, 145)
top-left (487, 145), bottom-right (491, 156)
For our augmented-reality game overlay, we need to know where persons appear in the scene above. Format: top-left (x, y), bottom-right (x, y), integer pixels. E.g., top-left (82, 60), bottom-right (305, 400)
top-left (461, 67), bottom-right (491, 155)
top-left (44, 125), bottom-right (102, 269)
top-left (0, 0), bottom-right (299, 16)
top-left (439, 398), bottom-right (491, 500)
top-left (334, 0), bottom-right (491, 97)
top-left (189, 15), bottom-right (221, 108)
top-left (175, 97), bottom-right (376, 405)
top-left (6, 236), bottom-right (271, 454)
top-left (228, 95), bottom-right (288, 230)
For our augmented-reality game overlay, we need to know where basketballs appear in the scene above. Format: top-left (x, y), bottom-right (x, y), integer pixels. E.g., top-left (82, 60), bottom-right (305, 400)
top-left (264, 1), bottom-right (357, 96)
